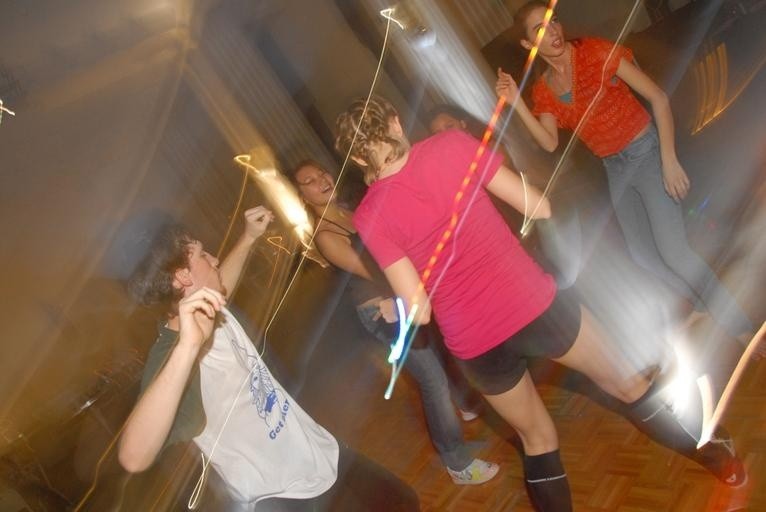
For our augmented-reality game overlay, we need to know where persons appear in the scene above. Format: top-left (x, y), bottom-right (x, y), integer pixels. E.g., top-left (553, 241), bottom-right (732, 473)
top-left (333, 96), bottom-right (751, 511)
top-left (426, 102), bottom-right (520, 256)
top-left (289, 159), bottom-right (501, 486)
top-left (493, 0), bottom-right (766, 362)
top-left (117, 206), bottom-right (423, 512)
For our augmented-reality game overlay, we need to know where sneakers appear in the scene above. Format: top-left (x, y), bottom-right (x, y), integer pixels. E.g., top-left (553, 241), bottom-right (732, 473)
top-left (462, 397), bottom-right (486, 421)
top-left (447, 459), bottom-right (500, 486)
top-left (714, 426), bottom-right (748, 487)
